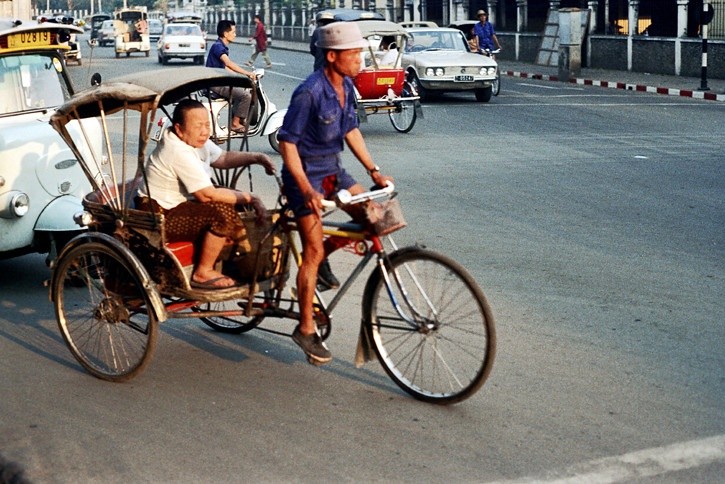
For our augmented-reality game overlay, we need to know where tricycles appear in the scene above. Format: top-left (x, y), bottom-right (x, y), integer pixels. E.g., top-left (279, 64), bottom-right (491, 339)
top-left (349, 20), bottom-right (426, 134)
top-left (42, 66), bottom-right (497, 405)
top-left (446, 20), bottom-right (502, 96)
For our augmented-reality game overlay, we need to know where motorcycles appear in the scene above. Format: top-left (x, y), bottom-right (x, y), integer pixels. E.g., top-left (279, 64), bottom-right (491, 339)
top-left (1, 15), bottom-right (112, 288)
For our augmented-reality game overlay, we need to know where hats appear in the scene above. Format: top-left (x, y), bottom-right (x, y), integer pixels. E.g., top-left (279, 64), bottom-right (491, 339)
top-left (477, 10), bottom-right (486, 14)
top-left (315, 21), bottom-right (369, 49)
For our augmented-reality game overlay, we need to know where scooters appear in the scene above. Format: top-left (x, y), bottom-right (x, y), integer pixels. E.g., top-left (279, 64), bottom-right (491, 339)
top-left (151, 59), bottom-right (290, 156)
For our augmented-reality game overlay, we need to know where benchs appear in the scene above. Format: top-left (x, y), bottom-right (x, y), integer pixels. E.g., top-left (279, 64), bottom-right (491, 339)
top-left (118, 35), bottom-right (124, 42)
top-left (140, 33), bottom-right (148, 41)
top-left (84, 173), bottom-right (262, 268)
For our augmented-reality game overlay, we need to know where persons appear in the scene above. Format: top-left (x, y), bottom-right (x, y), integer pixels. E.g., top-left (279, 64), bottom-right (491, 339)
top-left (276, 22), bottom-right (396, 364)
top-left (135, 99), bottom-right (273, 289)
top-left (206, 19), bottom-right (257, 133)
top-left (404, 33), bottom-right (417, 52)
top-left (244, 15), bottom-right (272, 68)
top-left (467, 9), bottom-right (502, 61)
top-left (370, 35), bottom-right (402, 69)
top-left (133, 17), bottom-right (146, 41)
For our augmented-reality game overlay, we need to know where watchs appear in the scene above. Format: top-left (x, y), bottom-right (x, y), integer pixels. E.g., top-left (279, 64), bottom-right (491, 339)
top-left (367, 164), bottom-right (381, 175)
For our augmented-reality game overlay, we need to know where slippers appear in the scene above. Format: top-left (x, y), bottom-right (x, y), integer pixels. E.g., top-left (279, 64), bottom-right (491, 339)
top-left (232, 126), bottom-right (246, 133)
top-left (190, 274), bottom-right (235, 289)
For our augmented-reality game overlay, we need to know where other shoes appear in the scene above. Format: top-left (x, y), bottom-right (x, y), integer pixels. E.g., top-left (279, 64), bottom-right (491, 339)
top-left (292, 326), bottom-right (332, 362)
top-left (244, 61), bottom-right (252, 66)
top-left (264, 65), bottom-right (271, 68)
top-left (299, 252), bottom-right (340, 289)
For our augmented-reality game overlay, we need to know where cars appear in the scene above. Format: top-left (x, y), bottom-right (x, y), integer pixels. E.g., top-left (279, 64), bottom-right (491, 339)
top-left (90, 11), bottom-right (209, 58)
top-left (400, 27), bottom-right (498, 102)
top-left (157, 22), bottom-right (208, 65)
top-left (398, 22), bottom-right (439, 28)
